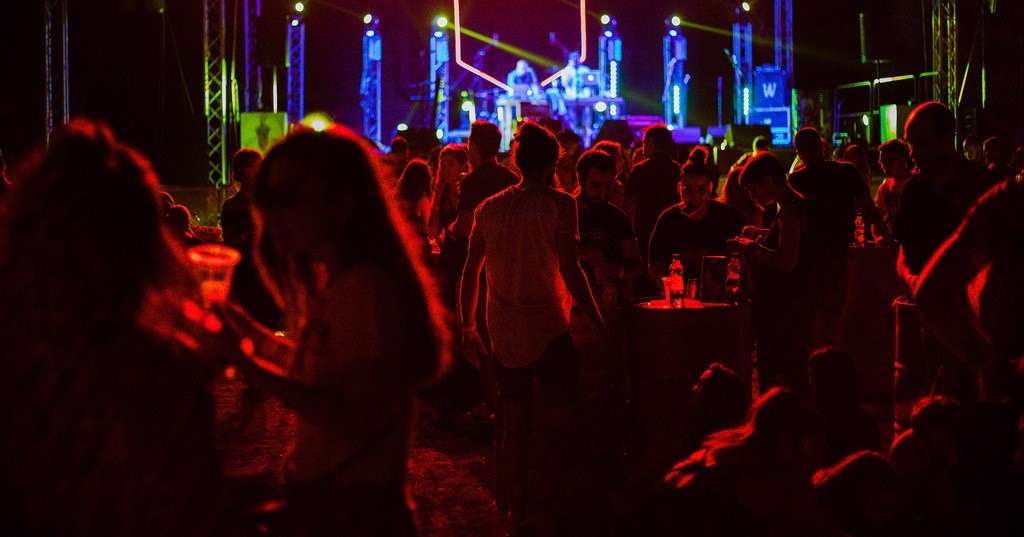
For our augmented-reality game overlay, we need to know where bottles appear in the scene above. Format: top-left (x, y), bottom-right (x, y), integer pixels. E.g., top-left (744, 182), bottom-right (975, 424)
top-left (854, 213), bottom-right (866, 245)
top-left (723, 252), bottom-right (741, 293)
top-left (668, 253), bottom-right (686, 308)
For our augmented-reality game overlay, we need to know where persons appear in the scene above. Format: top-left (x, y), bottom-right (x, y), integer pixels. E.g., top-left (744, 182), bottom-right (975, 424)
top-left (387, 95), bottom-right (1023, 537)
top-left (506, 59), bottom-right (539, 97)
top-left (163, 148), bottom-right (286, 332)
top-left (561, 53), bottom-right (593, 97)
top-left (0, 120), bottom-right (256, 536)
top-left (207, 112), bottom-right (451, 536)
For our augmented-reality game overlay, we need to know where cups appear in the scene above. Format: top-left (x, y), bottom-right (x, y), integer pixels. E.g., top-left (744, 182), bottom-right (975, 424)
top-left (660, 277), bottom-right (671, 300)
top-left (187, 242), bottom-right (241, 306)
